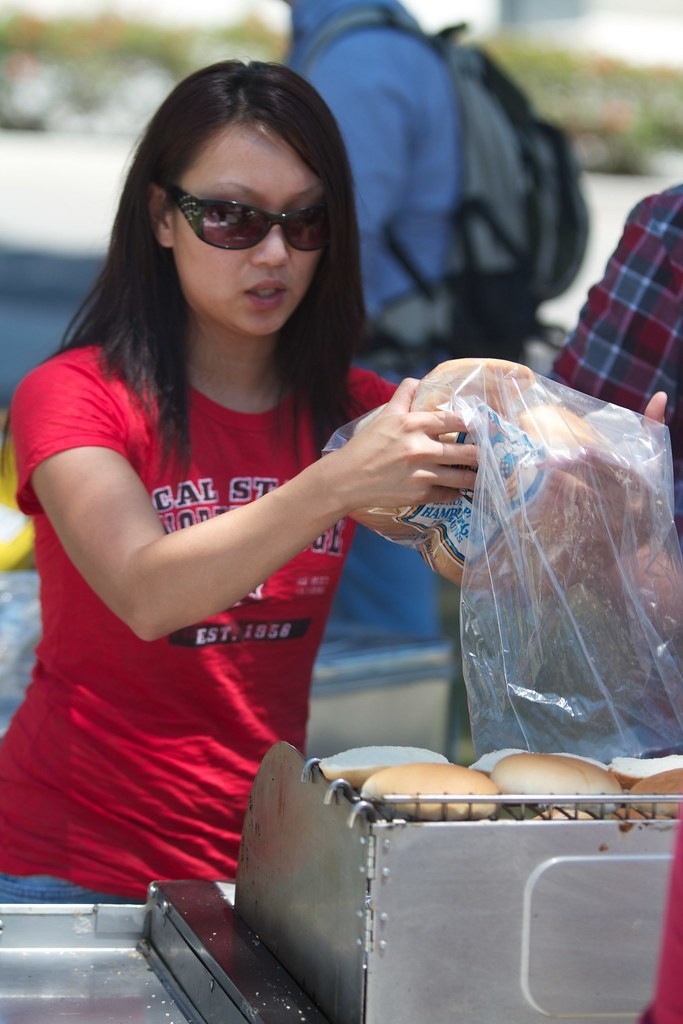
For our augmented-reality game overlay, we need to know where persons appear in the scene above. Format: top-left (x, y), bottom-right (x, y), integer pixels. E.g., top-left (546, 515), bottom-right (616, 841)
top-left (0, 59), bottom-right (478, 904)
top-left (280, 0), bottom-right (458, 640)
top-left (514, 182), bottom-right (683, 720)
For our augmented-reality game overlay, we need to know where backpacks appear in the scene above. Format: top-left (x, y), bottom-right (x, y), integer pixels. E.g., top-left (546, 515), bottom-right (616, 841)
top-left (298, 5), bottom-right (589, 373)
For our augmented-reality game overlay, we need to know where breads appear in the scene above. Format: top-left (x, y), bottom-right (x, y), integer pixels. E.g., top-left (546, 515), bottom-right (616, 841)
top-left (354, 358), bottom-right (606, 587)
top-left (319, 744), bottom-right (683, 821)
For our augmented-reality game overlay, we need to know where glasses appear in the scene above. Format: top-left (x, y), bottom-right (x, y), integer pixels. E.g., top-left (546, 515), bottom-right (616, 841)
top-left (156, 181), bottom-right (333, 251)
